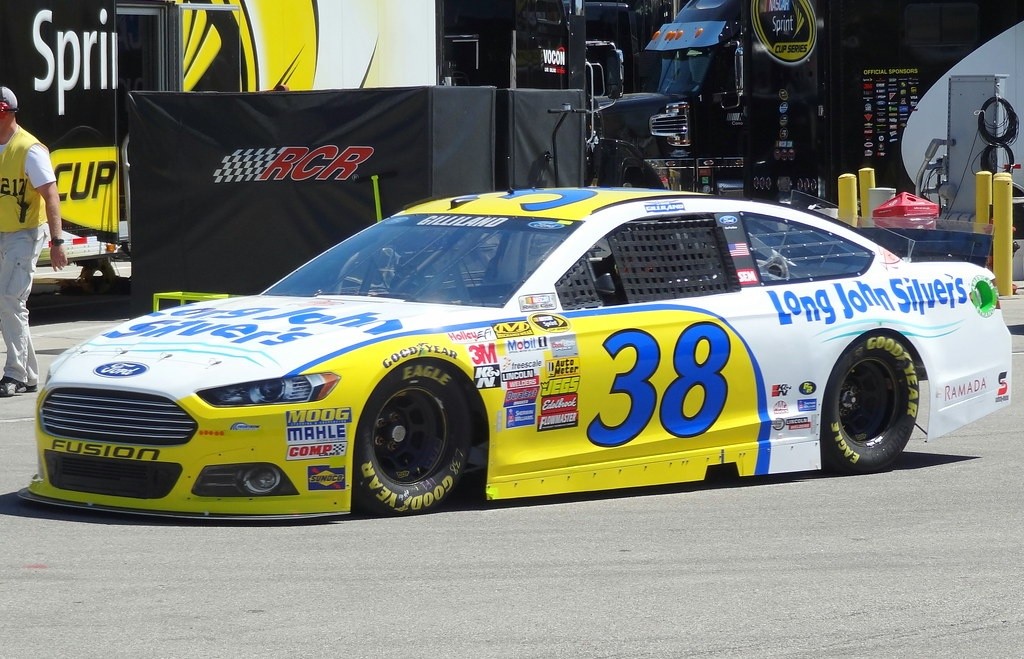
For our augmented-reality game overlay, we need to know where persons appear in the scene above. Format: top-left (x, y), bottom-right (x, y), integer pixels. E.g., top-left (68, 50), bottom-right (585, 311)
top-left (0, 85), bottom-right (68, 398)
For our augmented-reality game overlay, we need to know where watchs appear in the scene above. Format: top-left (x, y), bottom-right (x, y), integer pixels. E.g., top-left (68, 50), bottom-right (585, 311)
top-left (51, 237), bottom-right (65, 246)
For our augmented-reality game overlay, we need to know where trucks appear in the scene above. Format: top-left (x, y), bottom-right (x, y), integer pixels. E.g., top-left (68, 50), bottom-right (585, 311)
top-left (643, 0), bottom-right (1024, 203)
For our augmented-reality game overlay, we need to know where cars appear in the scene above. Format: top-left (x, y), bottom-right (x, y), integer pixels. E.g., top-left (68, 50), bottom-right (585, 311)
top-left (16, 185), bottom-right (1013, 520)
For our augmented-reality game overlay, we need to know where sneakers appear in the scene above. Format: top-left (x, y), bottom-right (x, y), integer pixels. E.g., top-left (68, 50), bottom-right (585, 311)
top-left (0, 375), bottom-right (38, 397)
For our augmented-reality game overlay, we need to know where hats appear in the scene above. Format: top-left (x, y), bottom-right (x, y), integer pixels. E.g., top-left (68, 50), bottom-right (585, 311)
top-left (0, 86), bottom-right (18, 110)
